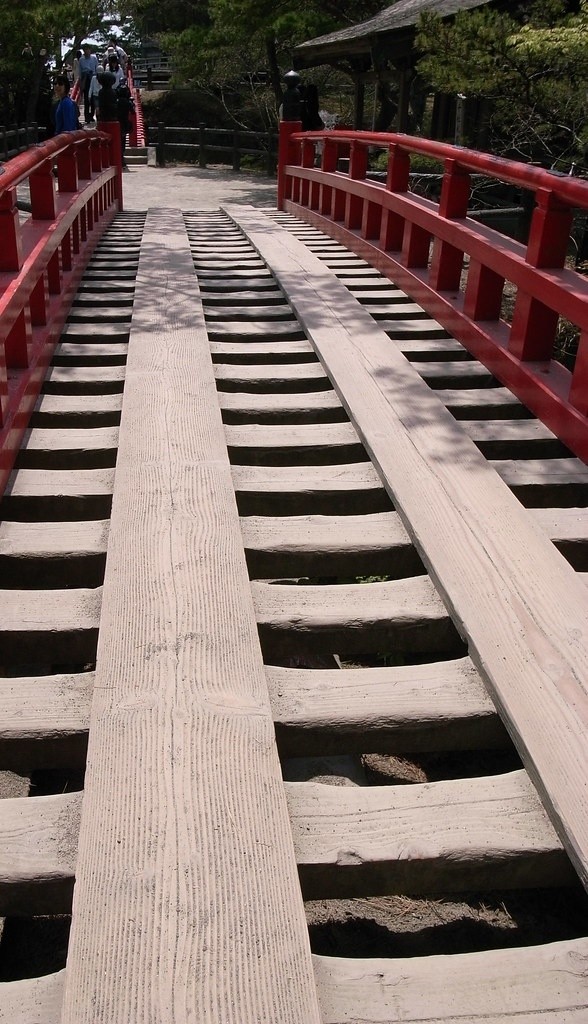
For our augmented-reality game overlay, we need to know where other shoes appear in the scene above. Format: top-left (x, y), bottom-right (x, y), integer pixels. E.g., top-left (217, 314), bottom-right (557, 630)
top-left (90, 116), bottom-right (95, 122)
top-left (122, 165), bottom-right (130, 173)
top-left (87, 120), bottom-right (90, 125)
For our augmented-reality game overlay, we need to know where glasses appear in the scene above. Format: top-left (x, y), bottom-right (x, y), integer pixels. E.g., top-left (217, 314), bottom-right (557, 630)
top-left (54, 81), bottom-right (64, 86)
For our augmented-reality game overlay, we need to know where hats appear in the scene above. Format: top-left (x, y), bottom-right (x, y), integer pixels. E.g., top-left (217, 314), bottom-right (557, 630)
top-left (96, 66), bottom-right (104, 74)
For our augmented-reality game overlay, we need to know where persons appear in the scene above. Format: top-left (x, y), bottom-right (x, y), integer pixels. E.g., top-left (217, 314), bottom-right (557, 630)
top-left (53, 74), bottom-right (80, 135)
top-left (73, 42), bottom-right (136, 173)
